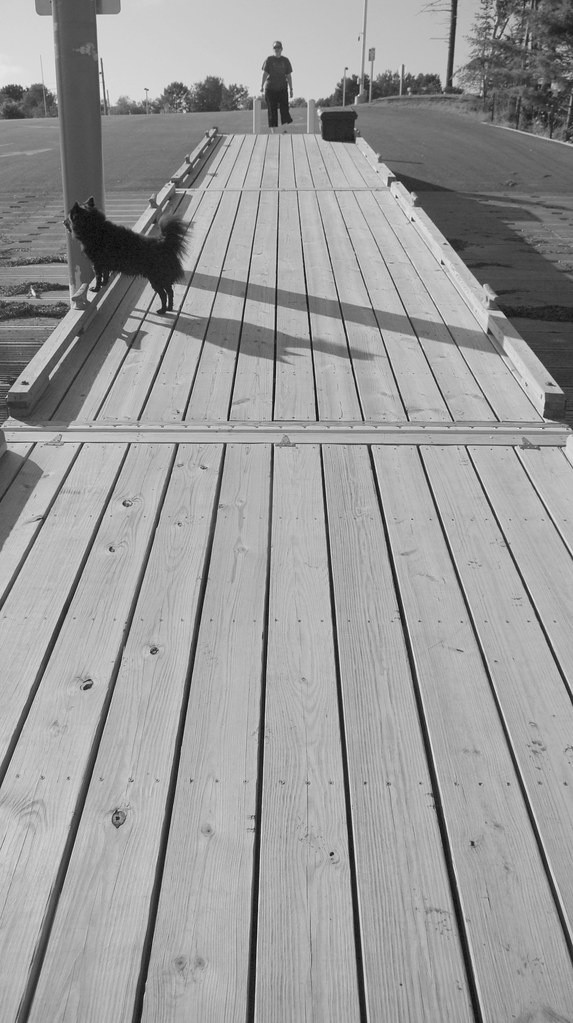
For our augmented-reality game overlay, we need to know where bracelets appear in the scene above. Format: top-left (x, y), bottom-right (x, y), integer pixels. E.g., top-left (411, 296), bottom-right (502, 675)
top-left (290, 88), bottom-right (293, 90)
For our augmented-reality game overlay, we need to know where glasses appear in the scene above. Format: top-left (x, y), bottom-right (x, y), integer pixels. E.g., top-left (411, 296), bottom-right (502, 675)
top-left (273, 46), bottom-right (282, 49)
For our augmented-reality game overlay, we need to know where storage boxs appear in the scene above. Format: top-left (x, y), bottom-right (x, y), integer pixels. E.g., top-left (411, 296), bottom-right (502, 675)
top-left (316, 106), bottom-right (358, 141)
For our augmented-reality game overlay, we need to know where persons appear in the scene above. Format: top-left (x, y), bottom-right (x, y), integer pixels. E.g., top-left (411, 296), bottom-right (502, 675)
top-left (259, 41), bottom-right (294, 134)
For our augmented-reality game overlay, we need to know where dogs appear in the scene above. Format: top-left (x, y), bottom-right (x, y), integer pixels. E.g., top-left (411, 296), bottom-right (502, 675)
top-left (62, 195), bottom-right (196, 315)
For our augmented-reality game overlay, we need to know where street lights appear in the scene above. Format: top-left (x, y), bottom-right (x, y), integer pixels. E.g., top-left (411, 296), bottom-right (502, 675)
top-left (144, 88), bottom-right (150, 116)
top-left (342, 67), bottom-right (349, 107)
